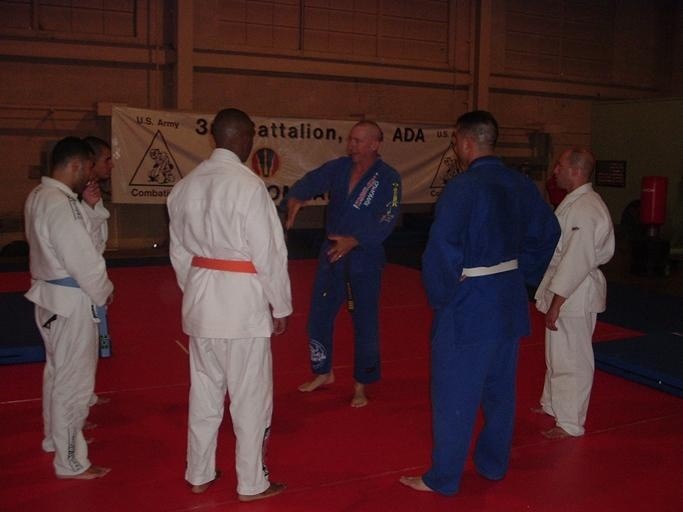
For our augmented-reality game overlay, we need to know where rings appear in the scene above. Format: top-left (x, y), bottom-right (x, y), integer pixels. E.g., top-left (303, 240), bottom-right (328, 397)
top-left (339, 255), bottom-right (342, 258)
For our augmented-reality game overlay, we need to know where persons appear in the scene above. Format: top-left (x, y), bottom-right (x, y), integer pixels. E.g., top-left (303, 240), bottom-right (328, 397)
top-left (399, 112), bottom-right (562, 496)
top-left (77, 137), bottom-right (115, 430)
top-left (23, 135), bottom-right (115, 480)
top-left (278, 120), bottom-right (401, 408)
top-left (166, 108), bottom-right (294, 501)
top-left (534, 145), bottom-right (616, 439)
